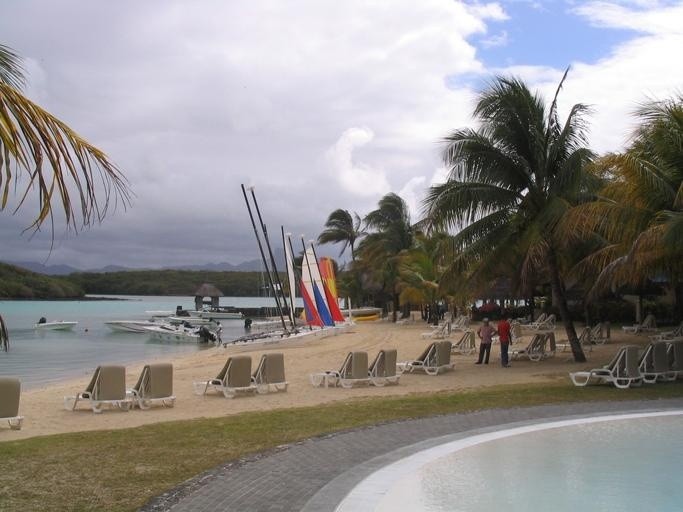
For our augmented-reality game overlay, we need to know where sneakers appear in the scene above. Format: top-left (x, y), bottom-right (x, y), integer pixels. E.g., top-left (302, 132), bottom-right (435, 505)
top-left (503, 364), bottom-right (512, 368)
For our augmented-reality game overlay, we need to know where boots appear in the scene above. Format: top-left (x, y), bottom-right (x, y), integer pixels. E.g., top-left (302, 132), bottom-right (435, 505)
top-left (484, 352), bottom-right (490, 364)
top-left (474, 352), bottom-right (484, 364)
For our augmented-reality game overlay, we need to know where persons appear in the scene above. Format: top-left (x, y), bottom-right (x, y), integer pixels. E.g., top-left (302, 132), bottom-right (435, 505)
top-left (199, 325), bottom-right (208, 343)
top-left (474, 316), bottom-right (497, 365)
top-left (243, 314), bottom-right (251, 329)
top-left (214, 321), bottom-right (223, 344)
top-left (496, 313), bottom-right (513, 369)
top-left (596, 304), bottom-right (611, 339)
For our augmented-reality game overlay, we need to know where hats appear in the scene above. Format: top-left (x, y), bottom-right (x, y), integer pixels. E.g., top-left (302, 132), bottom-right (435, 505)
top-left (482, 317), bottom-right (489, 322)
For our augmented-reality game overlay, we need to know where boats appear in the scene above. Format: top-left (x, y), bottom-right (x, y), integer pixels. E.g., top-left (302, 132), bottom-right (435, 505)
top-left (103, 307), bottom-right (242, 343)
top-left (29, 317), bottom-right (79, 330)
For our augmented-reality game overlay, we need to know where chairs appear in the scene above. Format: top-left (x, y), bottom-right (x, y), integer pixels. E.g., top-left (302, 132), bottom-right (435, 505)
top-left (399, 341), bottom-right (456, 375)
top-left (420, 311), bottom-right (683, 390)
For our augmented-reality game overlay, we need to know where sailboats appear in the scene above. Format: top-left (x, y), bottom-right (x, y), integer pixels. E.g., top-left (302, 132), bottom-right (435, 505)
top-left (220, 182), bottom-right (379, 354)
